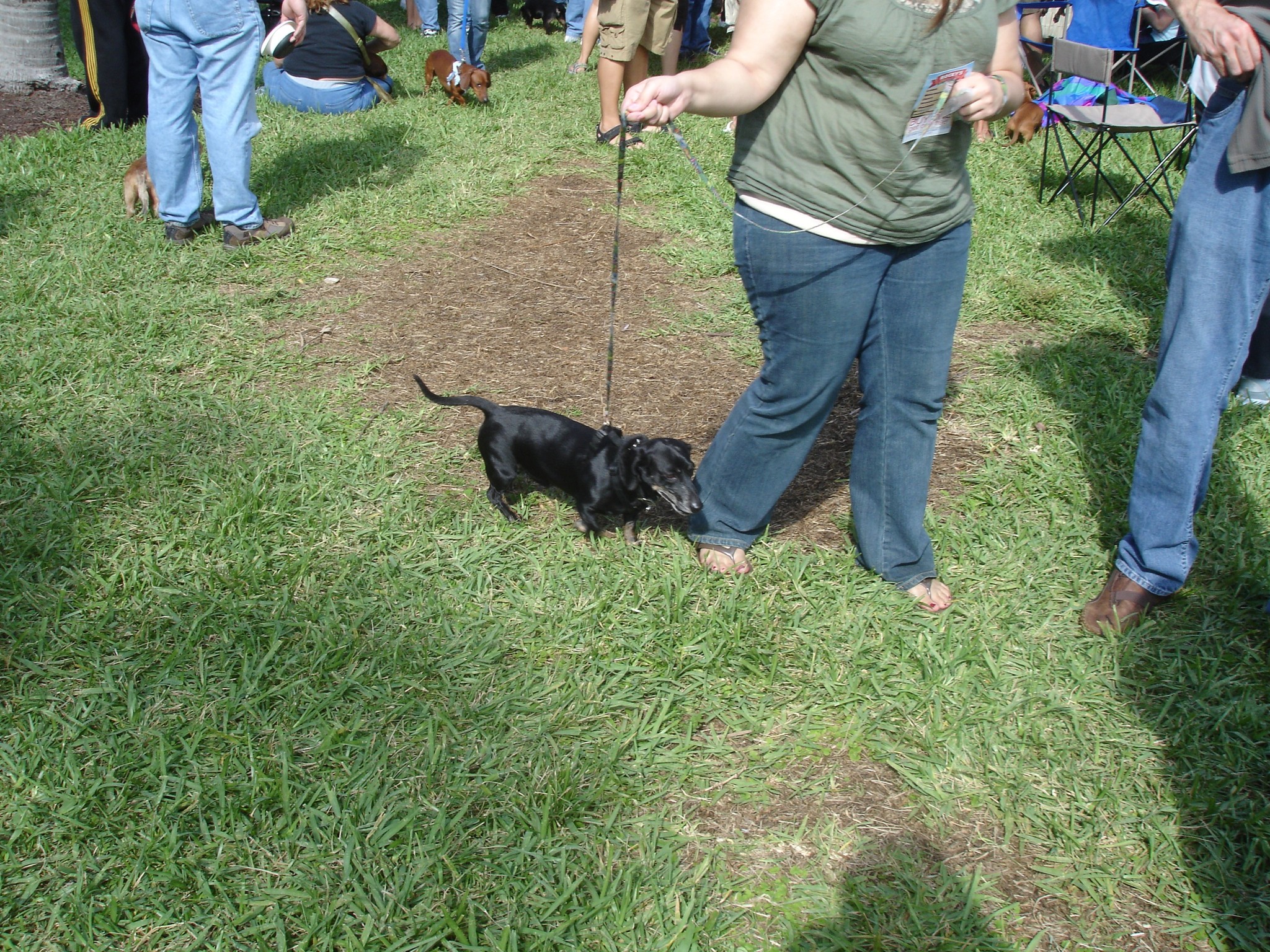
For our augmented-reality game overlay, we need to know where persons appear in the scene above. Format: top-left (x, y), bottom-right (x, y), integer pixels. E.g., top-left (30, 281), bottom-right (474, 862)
top-left (615, 0), bottom-right (1031, 612)
top-left (68, 0), bottom-right (150, 137)
top-left (551, 0), bottom-right (743, 149)
top-left (1013, 0), bottom-right (1187, 94)
top-left (1081, 0), bottom-right (1270, 638)
top-left (263, 0), bottom-right (402, 112)
top-left (400, 0), bottom-right (493, 81)
top-left (130, 0), bottom-right (313, 244)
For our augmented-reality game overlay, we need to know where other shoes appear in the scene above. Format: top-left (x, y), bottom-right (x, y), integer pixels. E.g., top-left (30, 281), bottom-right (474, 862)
top-left (688, 56), bottom-right (701, 62)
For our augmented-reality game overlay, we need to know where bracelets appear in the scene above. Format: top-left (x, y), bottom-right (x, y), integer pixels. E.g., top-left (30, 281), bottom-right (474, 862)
top-left (987, 74), bottom-right (1008, 117)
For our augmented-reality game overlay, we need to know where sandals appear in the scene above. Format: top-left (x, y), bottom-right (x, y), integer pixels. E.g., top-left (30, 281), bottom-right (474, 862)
top-left (692, 542), bottom-right (753, 577)
top-left (567, 63), bottom-right (587, 75)
top-left (906, 578), bottom-right (953, 615)
top-left (620, 117), bottom-right (644, 134)
top-left (596, 124), bottom-right (642, 150)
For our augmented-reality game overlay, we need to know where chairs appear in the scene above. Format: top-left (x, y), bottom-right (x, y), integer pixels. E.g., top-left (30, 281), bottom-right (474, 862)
top-left (1015, 0), bottom-right (1205, 238)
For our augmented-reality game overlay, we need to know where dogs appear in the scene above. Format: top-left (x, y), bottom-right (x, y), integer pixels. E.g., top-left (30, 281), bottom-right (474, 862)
top-left (425, 49), bottom-right (492, 107)
top-left (122, 152), bottom-right (159, 223)
top-left (1002, 84), bottom-right (1043, 147)
top-left (411, 372), bottom-right (705, 547)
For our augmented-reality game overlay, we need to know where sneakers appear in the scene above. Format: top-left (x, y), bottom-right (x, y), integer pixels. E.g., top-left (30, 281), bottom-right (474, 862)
top-left (224, 217), bottom-right (295, 249)
top-left (466, 27), bottom-right (470, 35)
top-left (1083, 569), bottom-right (1168, 639)
top-left (165, 211), bottom-right (219, 241)
top-left (423, 29), bottom-right (437, 38)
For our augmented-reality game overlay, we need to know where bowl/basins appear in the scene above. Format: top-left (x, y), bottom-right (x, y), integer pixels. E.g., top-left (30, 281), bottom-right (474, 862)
top-left (261, 10), bottom-right (281, 25)
top-left (261, 20), bottom-right (298, 59)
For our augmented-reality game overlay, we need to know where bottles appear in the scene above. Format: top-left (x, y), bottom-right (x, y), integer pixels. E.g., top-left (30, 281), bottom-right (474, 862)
top-left (265, 4), bottom-right (272, 15)
